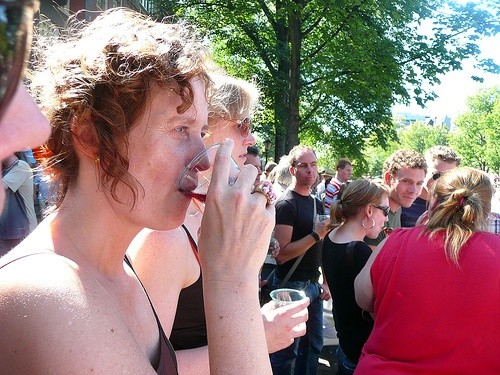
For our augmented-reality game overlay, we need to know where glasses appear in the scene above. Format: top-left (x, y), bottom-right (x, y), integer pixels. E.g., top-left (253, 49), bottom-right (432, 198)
top-left (0, 1), bottom-right (37, 122)
top-left (429, 172), bottom-right (440, 180)
top-left (375, 205), bottom-right (391, 216)
top-left (232, 117), bottom-right (251, 137)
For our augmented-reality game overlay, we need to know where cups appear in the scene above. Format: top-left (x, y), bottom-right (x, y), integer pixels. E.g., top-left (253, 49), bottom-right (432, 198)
top-left (269, 287), bottom-right (308, 312)
top-left (266, 230), bottom-right (277, 257)
top-left (319, 215), bottom-right (330, 231)
top-left (178, 142), bottom-right (245, 207)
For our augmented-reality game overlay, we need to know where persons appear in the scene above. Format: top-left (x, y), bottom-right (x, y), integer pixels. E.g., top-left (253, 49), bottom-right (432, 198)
top-left (0, 8), bottom-right (275, 375)
top-left (271, 143), bottom-right (330, 374)
top-left (398, 145), bottom-right (461, 228)
top-left (351, 167), bottom-right (500, 375)
top-left (258, 159), bottom-right (277, 180)
top-left (0, 0), bottom-right (53, 226)
top-left (0, 153), bottom-right (35, 257)
top-left (318, 167), bottom-right (336, 188)
top-left (321, 177), bottom-right (389, 374)
top-left (362, 147), bottom-right (427, 252)
top-left (322, 158), bottom-right (353, 218)
top-left (266, 154), bottom-right (292, 197)
top-left (119, 72), bottom-right (308, 373)
top-left (240, 141), bottom-right (279, 371)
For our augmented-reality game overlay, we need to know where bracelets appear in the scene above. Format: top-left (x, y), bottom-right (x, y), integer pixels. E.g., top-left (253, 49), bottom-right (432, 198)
top-left (310, 230), bottom-right (320, 242)
